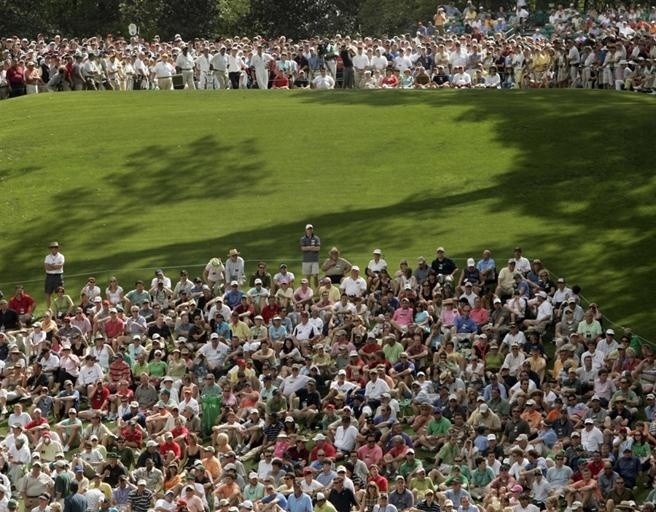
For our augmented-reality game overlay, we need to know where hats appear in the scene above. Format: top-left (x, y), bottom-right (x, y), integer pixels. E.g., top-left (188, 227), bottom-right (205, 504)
top-left (48, 242), bottom-right (59, 248)
top-left (10, 247), bottom-right (654, 510)
top-left (306, 224), bottom-right (313, 229)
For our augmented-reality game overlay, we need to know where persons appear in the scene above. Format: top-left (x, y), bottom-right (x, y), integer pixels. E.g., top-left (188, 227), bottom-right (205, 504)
top-left (1, 224), bottom-right (655, 511)
top-left (32, 2), bottom-right (656, 95)
